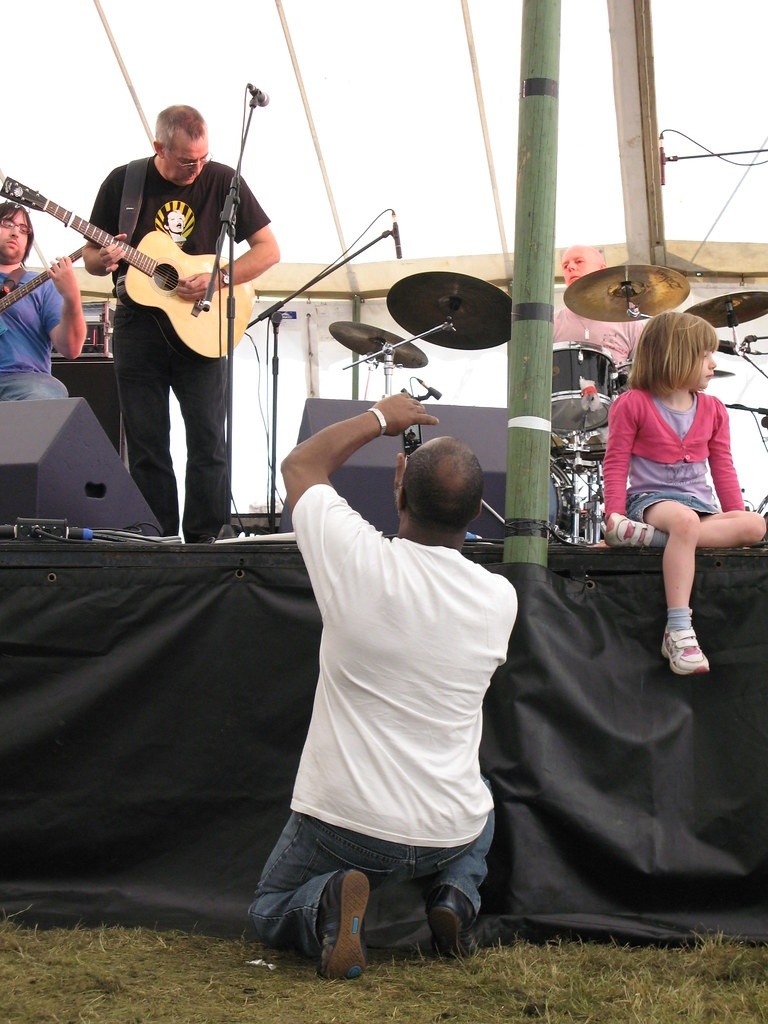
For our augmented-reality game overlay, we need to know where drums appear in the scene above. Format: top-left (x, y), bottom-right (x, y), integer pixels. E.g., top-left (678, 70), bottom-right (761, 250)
top-left (547, 448), bottom-right (576, 542)
top-left (581, 364), bottom-right (631, 460)
top-left (548, 339), bottom-right (615, 436)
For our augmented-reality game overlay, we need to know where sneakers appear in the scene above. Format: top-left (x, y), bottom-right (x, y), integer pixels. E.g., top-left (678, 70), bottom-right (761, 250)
top-left (605, 512), bottom-right (657, 549)
top-left (660, 627), bottom-right (710, 675)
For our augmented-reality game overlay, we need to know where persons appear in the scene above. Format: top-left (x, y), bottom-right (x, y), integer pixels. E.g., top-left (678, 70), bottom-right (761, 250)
top-left (0, 202), bottom-right (87, 401)
top-left (602, 312), bottom-right (766, 677)
top-left (551, 245), bottom-right (650, 446)
top-left (247, 393), bottom-right (518, 980)
top-left (82, 105), bottom-right (281, 543)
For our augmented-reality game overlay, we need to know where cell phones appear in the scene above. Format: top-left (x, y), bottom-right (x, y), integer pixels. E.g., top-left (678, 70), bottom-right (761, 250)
top-left (403, 424), bottom-right (423, 458)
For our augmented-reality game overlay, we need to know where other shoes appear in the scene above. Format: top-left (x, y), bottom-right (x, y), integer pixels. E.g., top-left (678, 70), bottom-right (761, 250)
top-left (213, 524), bottom-right (239, 542)
top-left (315, 868), bottom-right (368, 981)
top-left (424, 885), bottom-right (479, 962)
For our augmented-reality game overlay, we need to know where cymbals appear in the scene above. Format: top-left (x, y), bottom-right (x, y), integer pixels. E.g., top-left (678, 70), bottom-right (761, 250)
top-left (386, 271), bottom-right (513, 351)
top-left (328, 320), bottom-right (429, 368)
top-left (683, 290), bottom-right (768, 328)
top-left (563, 264), bottom-right (690, 322)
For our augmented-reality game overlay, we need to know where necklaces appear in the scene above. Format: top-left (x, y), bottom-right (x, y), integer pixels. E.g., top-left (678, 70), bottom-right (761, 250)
top-left (571, 311), bottom-right (593, 340)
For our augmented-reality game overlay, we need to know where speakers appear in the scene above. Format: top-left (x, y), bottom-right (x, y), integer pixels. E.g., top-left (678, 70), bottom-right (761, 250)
top-left (0, 397), bottom-right (165, 540)
top-left (277, 399), bottom-right (559, 543)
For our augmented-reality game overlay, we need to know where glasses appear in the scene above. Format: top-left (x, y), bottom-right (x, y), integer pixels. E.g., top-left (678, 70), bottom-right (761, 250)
top-left (166, 150), bottom-right (214, 170)
top-left (1, 217), bottom-right (33, 235)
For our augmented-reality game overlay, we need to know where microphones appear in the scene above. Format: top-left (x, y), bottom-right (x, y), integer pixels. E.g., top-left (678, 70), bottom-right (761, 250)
top-left (247, 83), bottom-right (269, 107)
top-left (659, 134), bottom-right (665, 185)
top-left (416, 379), bottom-right (442, 401)
top-left (392, 211), bottom-right (402, 258)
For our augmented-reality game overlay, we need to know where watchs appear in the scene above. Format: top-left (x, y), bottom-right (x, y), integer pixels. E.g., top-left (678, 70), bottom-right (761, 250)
top-left (219, 269), bottom-right (230, 288)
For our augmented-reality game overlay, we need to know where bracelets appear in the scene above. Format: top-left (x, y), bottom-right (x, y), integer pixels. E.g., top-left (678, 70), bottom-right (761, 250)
top-left (366, 407), bottom-right (387, 436)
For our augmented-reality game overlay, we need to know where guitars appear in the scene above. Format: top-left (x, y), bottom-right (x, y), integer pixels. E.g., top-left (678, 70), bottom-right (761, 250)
top-left (0, 244), bottom-right (87, 315)
top-left (0, 176), bottom-right (256, 361)
